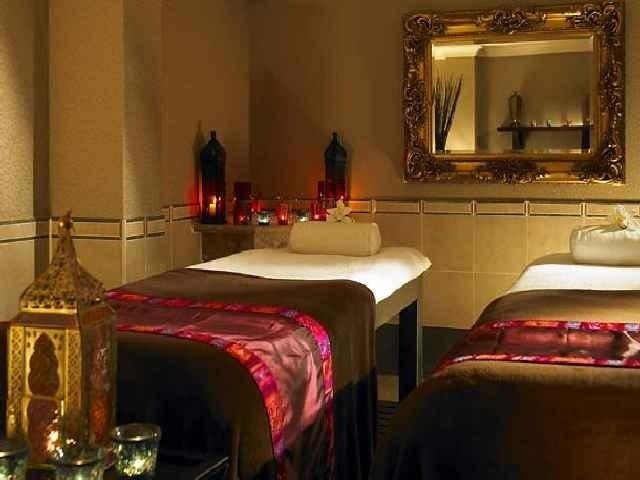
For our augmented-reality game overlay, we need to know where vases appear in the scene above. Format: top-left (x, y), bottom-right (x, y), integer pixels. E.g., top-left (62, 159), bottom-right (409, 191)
top-left (508, 92), bottom-right (521, 122)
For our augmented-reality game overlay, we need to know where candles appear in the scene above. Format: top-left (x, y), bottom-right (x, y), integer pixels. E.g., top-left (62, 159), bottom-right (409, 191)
top-left (208, 196), bottom-right (216, 216)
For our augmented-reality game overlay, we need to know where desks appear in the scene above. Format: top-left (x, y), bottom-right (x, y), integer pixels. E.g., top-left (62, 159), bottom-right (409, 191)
top-left (26, 446), bottom-right (232, 480)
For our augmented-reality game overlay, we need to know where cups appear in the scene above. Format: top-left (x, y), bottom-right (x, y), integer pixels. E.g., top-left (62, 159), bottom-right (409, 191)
top-left (110, 422), bottom-right (162, 480)
top-left (527, 118), bottom-right (576, 128)
top-left (229, 191), bottom-right (328, 226)
top-left (0, 438), bottom-right (29, 480)
top-left (48, 445), bottom-right (105, 480)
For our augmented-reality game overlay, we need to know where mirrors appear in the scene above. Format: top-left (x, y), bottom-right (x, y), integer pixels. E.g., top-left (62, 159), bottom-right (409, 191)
top-left (401, 0), bottom-right (626, 187)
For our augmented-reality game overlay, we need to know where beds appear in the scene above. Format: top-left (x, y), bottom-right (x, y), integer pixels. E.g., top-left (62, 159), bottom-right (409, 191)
top-left (388, 252), bottom-right (640, 478)
top-left (101, 242), bottom-right (432, 477)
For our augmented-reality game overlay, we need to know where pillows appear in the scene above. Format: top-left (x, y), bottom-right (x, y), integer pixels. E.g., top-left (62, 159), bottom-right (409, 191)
top-left (288, 219), bottom-right (381, 257)
top-left (569, 223), bottom-right (640, 264)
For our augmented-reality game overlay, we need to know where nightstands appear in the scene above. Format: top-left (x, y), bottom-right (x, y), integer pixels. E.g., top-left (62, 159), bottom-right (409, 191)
top-left (193, 222), bottom-right (292, 261)
